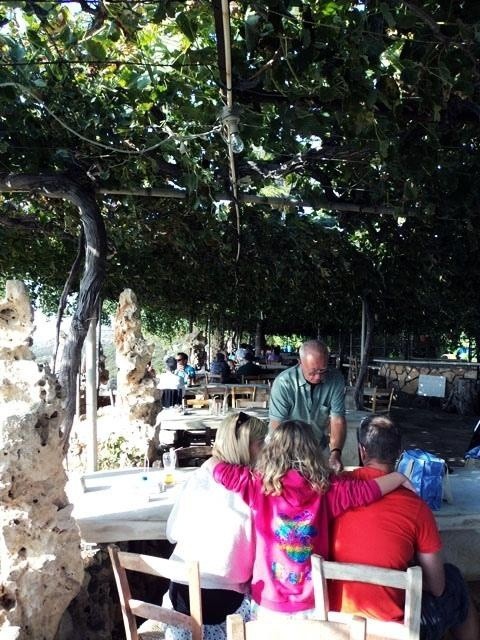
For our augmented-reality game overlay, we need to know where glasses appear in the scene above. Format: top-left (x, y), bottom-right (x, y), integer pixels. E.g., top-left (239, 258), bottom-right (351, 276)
top-left (236, 413), bottom-right (249, 439)
top-left (306, 368), bottom-right (326, 375)
top-left (177, 359), bottom-right (183, 362)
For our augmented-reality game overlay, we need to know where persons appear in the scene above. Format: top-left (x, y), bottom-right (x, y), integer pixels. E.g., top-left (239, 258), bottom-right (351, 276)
top-left (203, 419), bottom-right (418, 624)
top-left (269, 339), bottom-right (347, 473)
top-left (147, 343), bottom-right (280, 408)
top-left (323, 412), bottom-right (479, 640)
top-left (165, 412), bottom-right (270, 640)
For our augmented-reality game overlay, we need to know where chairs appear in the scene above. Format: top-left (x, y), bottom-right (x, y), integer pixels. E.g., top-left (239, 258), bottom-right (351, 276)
top-left (155, 344), bottom-right (396, 446)
top-left (174, 447), bottom-right (220, 465)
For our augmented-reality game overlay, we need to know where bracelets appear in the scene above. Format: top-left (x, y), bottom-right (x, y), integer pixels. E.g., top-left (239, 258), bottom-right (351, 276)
top-left (330, 448), bottom-right (342, 456)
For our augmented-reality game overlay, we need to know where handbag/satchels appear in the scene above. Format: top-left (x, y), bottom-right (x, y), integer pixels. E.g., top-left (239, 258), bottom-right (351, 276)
top-left (395, 449), bottom-right (453, 510)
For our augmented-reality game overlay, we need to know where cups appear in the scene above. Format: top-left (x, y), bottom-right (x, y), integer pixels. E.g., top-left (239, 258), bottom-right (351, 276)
top-left (186, 372), bottom-right (212, 388)
top-left (160, 396), bottom-right (229, 420)
top-left (137, 451), bottom-right (178, 494)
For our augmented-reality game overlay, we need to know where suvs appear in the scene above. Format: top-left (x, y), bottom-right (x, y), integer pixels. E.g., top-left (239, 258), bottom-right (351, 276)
top-left (79, 375), bottom-right (87, 392)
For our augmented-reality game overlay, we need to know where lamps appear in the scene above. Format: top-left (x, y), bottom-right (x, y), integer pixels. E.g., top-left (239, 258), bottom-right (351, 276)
top-left (221, 106), bottom-right (245, 154)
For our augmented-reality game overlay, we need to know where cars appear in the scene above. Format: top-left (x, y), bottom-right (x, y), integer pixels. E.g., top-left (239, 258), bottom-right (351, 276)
top-left (441, 349), bottom-right (462, 360)
top-left (97, 384), bottom-right (108, 392)
